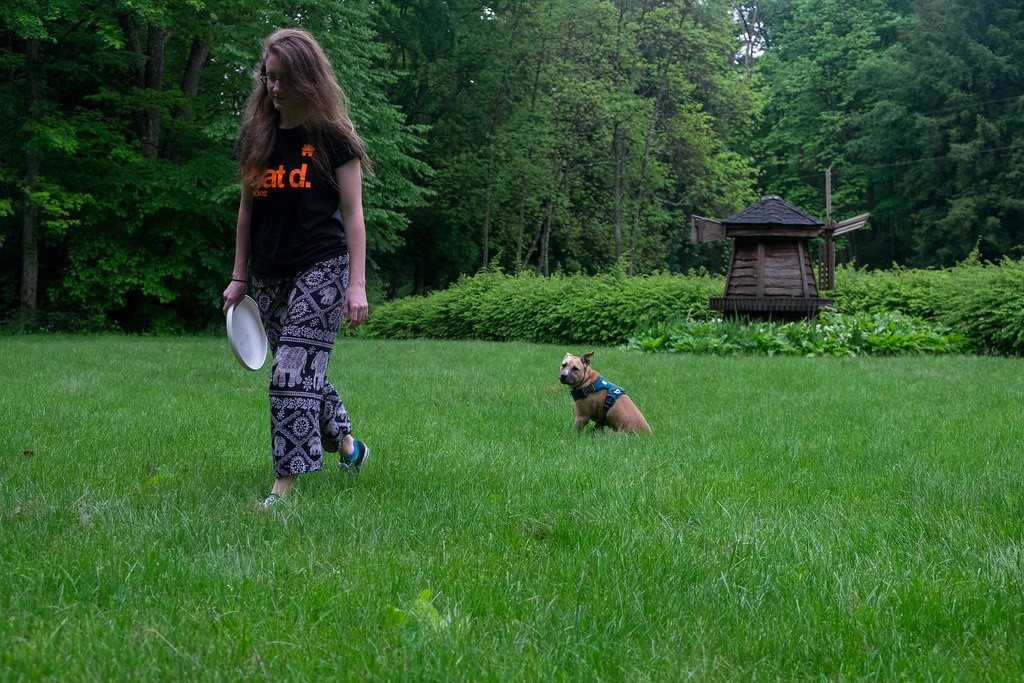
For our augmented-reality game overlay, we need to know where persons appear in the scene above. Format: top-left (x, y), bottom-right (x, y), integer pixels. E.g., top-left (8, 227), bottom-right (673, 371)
top-left (222, 28), bottom-right (371, 511)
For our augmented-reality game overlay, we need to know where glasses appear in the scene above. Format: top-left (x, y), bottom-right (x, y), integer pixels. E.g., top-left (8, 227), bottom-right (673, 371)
top-left (260, 75), bottom-right (295, 86)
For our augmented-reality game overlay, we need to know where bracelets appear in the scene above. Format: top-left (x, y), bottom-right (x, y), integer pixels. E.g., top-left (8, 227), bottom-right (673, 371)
top-left (230, 278), bottom-right (249, 283)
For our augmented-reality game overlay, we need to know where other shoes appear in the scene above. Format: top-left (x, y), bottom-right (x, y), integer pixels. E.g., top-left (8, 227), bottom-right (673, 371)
top-left (338, 437), bottom-right (369, 475)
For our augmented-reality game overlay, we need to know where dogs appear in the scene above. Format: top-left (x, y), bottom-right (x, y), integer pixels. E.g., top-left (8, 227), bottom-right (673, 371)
top-left (559, 349), bottom-right (652, 436)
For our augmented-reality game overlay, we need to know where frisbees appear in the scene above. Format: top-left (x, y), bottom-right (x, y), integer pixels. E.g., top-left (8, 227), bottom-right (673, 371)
top-left (226, 294), bottom-right (268, 372)
